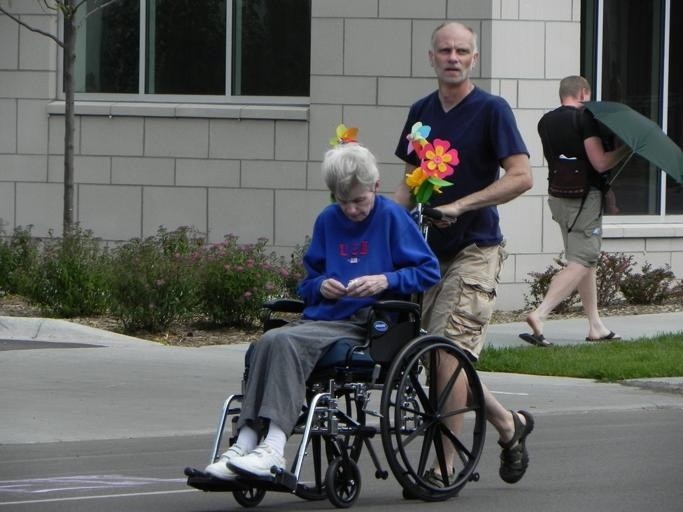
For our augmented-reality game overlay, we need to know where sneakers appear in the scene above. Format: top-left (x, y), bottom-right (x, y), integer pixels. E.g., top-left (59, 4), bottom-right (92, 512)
top-left (227, 449), bottom-right (285, 475)
top-left (205, 443), bottom-right (251, 481)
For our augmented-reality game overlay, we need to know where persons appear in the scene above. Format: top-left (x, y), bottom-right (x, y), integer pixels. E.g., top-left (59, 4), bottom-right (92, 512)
top-left (603, 134), bottom-right (627, 212)
top-left (393, 22), bottom-right (534, 500)
top-left (520, 76), bottom-right (622, 347)
top-left (206, 143), bottom-right (441, 480)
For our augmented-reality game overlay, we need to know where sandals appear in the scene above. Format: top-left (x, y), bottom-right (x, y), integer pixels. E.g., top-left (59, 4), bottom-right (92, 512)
top-left (402, 467), bottom-right (458, 501)
top-left (498, 410), bottom-right (534, 484)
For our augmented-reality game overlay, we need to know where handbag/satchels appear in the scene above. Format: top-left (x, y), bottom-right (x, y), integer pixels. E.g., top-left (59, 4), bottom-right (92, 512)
top-left (541, 115), bottom-right (591, 198)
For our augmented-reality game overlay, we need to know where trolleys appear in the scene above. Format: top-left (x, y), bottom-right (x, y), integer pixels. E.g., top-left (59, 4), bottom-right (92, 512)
top-left (188, 202), bottom-right (486, 507)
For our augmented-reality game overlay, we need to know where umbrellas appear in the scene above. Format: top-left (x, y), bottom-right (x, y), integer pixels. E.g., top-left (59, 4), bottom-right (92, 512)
top-left (581, 101), bottom-right (683, 197)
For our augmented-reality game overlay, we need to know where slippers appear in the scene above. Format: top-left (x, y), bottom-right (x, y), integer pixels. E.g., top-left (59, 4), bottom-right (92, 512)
top-left (586, 332), bottom-right (621, 342)
top-left (520, 332), bottom-right (553, 348)
